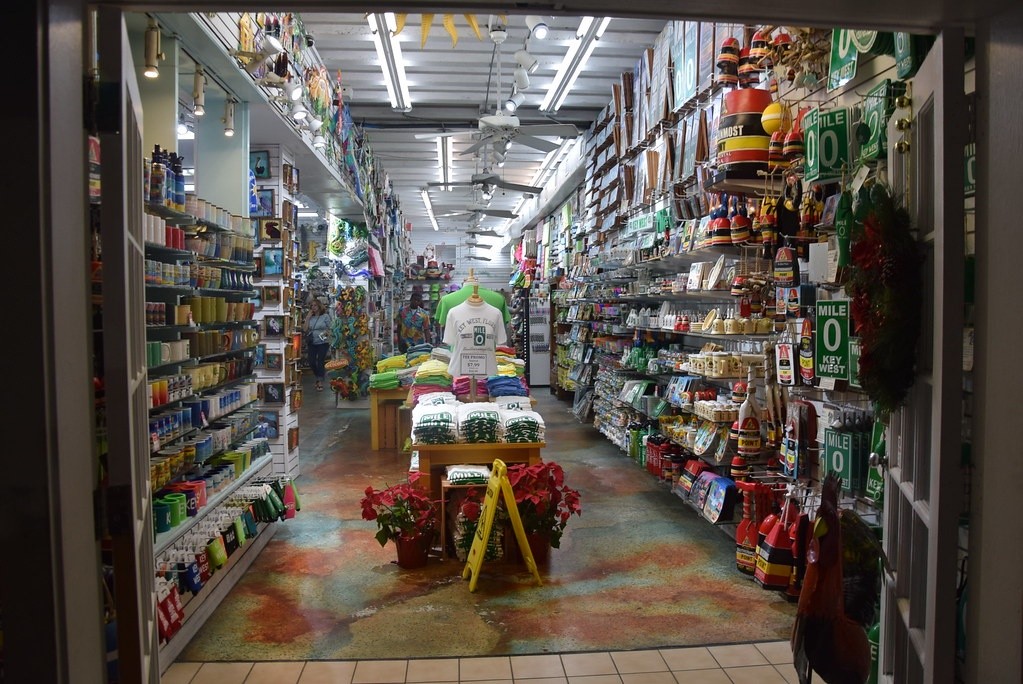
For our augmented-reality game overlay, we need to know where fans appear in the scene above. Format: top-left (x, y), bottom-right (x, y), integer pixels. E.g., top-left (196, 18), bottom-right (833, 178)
top-left (447, 183), bottom-right (519, 263)
top-left (428, 112), bottom-right (543, 195)
top-left (415, 25), bottom-right (579, 154)
top-left (450, 157), bottom-right (519, 218)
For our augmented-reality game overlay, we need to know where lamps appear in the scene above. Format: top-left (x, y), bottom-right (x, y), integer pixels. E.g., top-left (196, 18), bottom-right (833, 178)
top-left (254, 72), bottom-right (303, 102)
top-left (526, 15), bottom-right (549, 41)
top-left (365, 13), bottom-right (413, 113)
top-left (421, 188), bottom-right (439, 231)
top-left (537, 16), bottom-right (612, 115)
top-left (305, 110), bottom-right (323, 134)
top-left (268, 95), bottom-right (309, 120)
top-left (296, 114), bottom-right (327, 148)
top-left (192, 64), bottom-right (205, 115)
top-left (221, 94), bottom-right (235, 136)
top-left (436, 129), bottom-right (452, 192)
top-left (143, 16), bottom-right (166, 78)
top-left (513, 65), bottom-right (531, 90)
top-left (487, 139), bottom-right (512, 168)
top-left (514, 49), bottom-right (539, 73)
top-left (229, 35), bottom-right (284, 74)
top-left (505, 83), bottom-right (526, 112)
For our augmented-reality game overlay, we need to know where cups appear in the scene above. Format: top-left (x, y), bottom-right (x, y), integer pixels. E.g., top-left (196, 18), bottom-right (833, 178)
top-left (141, 158), bottom-right (269, 546)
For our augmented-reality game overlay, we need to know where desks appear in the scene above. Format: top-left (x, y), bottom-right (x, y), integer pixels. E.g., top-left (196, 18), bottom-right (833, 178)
top-left (366, 387), bottom-right (547, 565)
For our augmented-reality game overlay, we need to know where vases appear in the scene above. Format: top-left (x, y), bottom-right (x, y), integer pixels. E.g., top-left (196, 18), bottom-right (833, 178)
top-left (517, 530), bottom-right (549, 566)
top-left (395, 531), bottom-right (434, 569)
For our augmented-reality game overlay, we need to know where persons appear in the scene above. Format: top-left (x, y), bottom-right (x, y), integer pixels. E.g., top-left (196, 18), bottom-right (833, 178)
top-left (395, 292), bottom-right (431, 355)
top-left (303, 300), bottom-right (332, 391)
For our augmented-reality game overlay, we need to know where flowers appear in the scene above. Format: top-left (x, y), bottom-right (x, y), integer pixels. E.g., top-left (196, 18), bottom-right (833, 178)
top-left (360, 470), bottom-right (448, 548)
top-left (463, 461), bottom-right (582, 549)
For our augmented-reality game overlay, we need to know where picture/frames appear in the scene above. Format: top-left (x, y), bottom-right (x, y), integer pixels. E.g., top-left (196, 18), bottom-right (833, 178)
top-left (250, 411), bottom-right (280, 439)
top-left (250, 150), bottom-right (271, 179)
top-left (248, 163), bottom-right (303, 411)
top-left (288, 426), bottom-right (300, 450)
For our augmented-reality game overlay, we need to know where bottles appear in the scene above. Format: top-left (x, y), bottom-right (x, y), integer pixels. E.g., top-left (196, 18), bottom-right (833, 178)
top-left (736, 388), bottom-right (761, 457)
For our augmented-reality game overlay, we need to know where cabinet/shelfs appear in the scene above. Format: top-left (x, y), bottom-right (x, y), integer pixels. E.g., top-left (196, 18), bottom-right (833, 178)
top-left (88, 158), bottom-right (281, 684)
top-left (552, 15), bottom-right (787, 541)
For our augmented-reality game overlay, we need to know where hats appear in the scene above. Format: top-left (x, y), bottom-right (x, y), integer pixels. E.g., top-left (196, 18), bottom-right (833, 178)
top-left (404, 283), bottom-right (462, 301)
